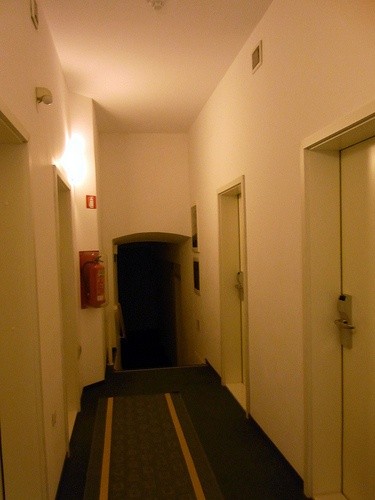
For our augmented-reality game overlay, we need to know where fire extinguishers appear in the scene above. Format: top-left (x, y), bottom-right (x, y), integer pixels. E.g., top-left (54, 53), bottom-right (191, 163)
top-left (84, 255), bottom-right (107, 307)
